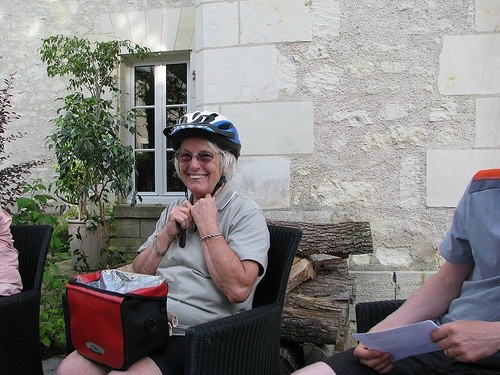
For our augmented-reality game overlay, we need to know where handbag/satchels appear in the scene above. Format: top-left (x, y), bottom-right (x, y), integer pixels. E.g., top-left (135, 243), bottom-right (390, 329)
top-left (62, 269), bottom-right (168, 371)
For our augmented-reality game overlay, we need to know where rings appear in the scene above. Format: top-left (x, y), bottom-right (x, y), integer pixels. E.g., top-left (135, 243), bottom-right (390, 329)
top-left (446, 349), bottom-right (450, 356)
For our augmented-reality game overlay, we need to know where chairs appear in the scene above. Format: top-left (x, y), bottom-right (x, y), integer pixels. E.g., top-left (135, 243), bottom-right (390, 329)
top-left (0, 224), bottom-right (55, 375)
top-left (157, 225), bottom-right (302, 375)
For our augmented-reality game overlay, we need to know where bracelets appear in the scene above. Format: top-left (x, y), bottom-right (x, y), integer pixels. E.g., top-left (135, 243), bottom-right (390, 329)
top-left (153, 233), bottom-right (169, 256)
top-left (200, 232), bottom-right (222, 241)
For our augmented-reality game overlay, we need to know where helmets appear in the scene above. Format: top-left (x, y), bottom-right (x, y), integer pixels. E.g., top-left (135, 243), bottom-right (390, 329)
top-left (163, 111), bottom-right (241, 159)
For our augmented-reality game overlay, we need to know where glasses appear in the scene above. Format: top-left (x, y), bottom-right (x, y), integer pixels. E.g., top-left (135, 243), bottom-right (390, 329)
top-left (174, 151), bottom-right (221, 163)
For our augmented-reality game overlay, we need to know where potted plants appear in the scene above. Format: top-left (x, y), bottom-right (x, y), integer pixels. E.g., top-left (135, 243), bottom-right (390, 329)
top-left (36, 34), bottom-right (162, 273)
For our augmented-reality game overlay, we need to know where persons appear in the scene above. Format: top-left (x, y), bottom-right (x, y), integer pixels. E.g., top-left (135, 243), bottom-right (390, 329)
top-left (0, 206), bottom-right (24, 296)
top-left (54, 110), bottom-right (270, 375)
top-left (290, 169), bottom-right (500, 375)
